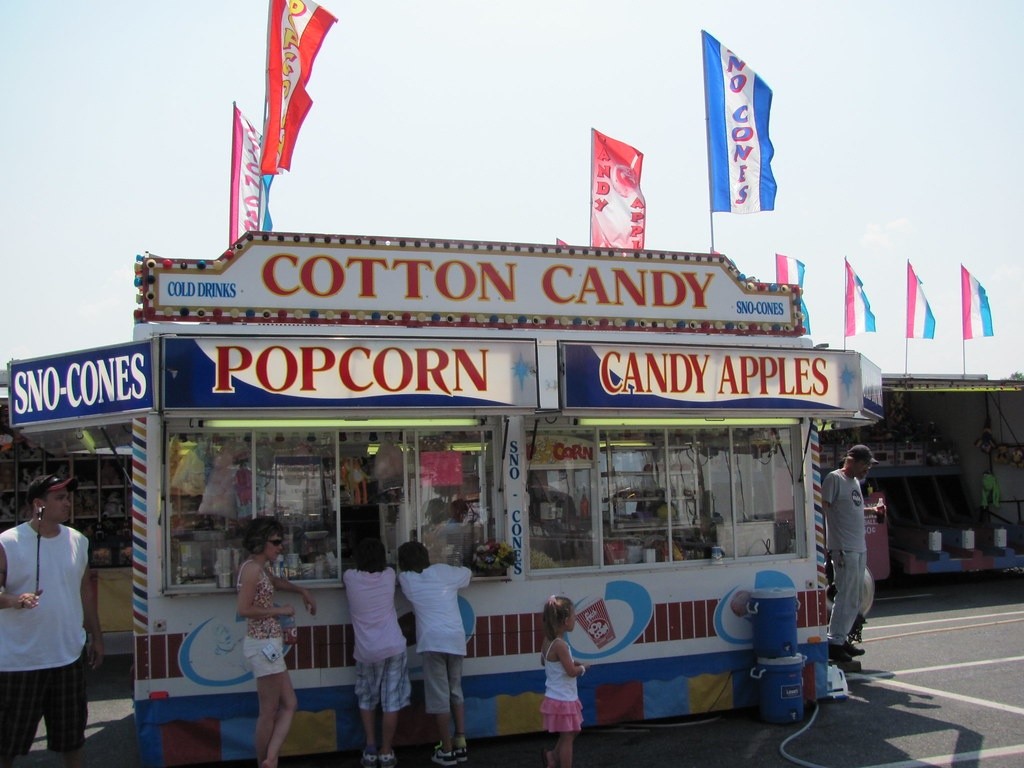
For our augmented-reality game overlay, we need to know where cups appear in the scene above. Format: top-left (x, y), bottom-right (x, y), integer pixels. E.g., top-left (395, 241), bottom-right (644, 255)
top-left (301, 563), bottom-right (315, 579)
top-left (712, 547), bottom-right (723, 564)
top-left (272, 554), bottom-right (284, 579)
top-left (287, 553), bottom-right (300, 580)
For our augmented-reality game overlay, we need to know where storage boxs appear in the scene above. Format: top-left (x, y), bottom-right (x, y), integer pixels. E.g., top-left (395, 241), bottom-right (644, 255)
top-left (895, 443), bottom-right (924, 464)
top-left (835, 444), bottom-right (861, 466)
top-left (867, 442), bottom-right (895, 465)
top-left (819, 444), bottom-right (835, 468)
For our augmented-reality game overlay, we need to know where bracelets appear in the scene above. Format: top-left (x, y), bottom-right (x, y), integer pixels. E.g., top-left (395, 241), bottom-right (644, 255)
top-left (580, 665), bottom-right (586, 677)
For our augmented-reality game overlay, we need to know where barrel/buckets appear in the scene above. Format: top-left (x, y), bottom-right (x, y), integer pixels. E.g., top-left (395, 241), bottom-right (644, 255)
top-left (750, 652), bottom-right (807, 724)
top-left (746, 587), bottom-right (799, 660)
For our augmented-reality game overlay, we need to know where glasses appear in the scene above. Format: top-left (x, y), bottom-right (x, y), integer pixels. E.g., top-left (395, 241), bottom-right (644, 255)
top-left (266, 538), bottom-right (284, 546)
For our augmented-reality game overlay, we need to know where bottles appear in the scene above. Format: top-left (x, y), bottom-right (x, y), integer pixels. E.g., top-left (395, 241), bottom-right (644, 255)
top-left (876, 497), bottom-right (884, 523)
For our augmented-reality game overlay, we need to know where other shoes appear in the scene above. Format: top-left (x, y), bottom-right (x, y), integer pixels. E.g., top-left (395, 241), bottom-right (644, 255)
top-left (542, 748), bottom-right (561, 768)
top-left (829, 644), bottom-right (852, 662)
top-left (843, 641), bottom-right (865, 656)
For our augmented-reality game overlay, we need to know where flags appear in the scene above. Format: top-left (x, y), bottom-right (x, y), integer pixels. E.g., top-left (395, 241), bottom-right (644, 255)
top-left (776, 254), bottom-right (811, 335)
top-left (704, 31), bottom-right (778, 213)
top-left (908, 263), bottom-right (936, 339)
top-left (230, 109), bottom-right (272, 250)
top-left (593, 130), bottom-right (646, 251)
top-left (263, 0), bottom-right (338, 176)
top-left (961, 265), bottom-right (995, 341)
top-left (846, 260), bottom-right (877, 336)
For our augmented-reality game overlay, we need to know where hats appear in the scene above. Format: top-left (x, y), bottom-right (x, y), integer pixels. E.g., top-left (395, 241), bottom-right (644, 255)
top-left (847, 445), bottom-right (879, 465)
top-left (29, 475), bottom-right (78, 504)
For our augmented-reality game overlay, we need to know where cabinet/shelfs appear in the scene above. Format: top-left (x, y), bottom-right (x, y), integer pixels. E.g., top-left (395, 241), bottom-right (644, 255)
top-left (600, 430), bottom-right (701, 533)
top-left (0, 453), bottom-right (133, 531)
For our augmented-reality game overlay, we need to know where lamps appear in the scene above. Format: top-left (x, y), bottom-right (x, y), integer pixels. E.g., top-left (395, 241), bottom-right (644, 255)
top-left (366, 442), bottom-right (489, 455)
top-left (385, 432), bottom-right (393, 441)
top-left (599, 440), bottom-right (656, 448)
top-left (458, 432), bottom-right (468, 439)
top-left (275, 432), bottom-right (285, 443)
top-left (525, 427), bottom-right (754, 440)
top-left (198, 418), bottom-right (485, 428)
top-left (306, 432), bottom-right (317, 442)
top-left (179, 433), bottom-right (188, 442)
top-left (369, 432), bottom-right (378, 441)
top-left (444, 431), bottom-right (453, 439)
top-left (195, 433), bottom-right (204, 443)
top-left (573, 417), bottom-right (804, 426)
top-left (244, 432), bottom-right (252, 442)
top-left (260, 432), bottom-right (270, 443)
top-left (76, 428), bottom-right (97, 455)
top-left (353, 432), bottom-right (362, 441)
top-left (291, 432), bottom-right (300, 442)
top-left (399, 432), bottom-right (408, 441)
top-left (339, 432), bottom-right (347, 442)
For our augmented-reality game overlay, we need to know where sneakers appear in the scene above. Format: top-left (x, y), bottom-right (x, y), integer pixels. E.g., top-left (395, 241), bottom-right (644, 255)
top-left (360, 750), bottom-right (379, 768)
top-left (431, 741), bottom-right (458, 766)
top-left (455, 746), bottom-right (468, 761)
top-left (378, 750), bottom-right (398, 768)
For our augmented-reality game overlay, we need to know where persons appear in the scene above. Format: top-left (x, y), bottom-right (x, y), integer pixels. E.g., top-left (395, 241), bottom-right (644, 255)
top-left (343, 538), bottom-right (412, 768)
top-left (397, 541), bottom-right (474, 765)
top-left (234, 518), bottom-right (317, 768)
top-left (0, 475), bottom-right (105, 768)
top-left (541, 595), bottom-right (590, 768)
top-left (424, 499), bottom-right (469, 558)
top-left (822, 444), bottom-right (887, 664)
